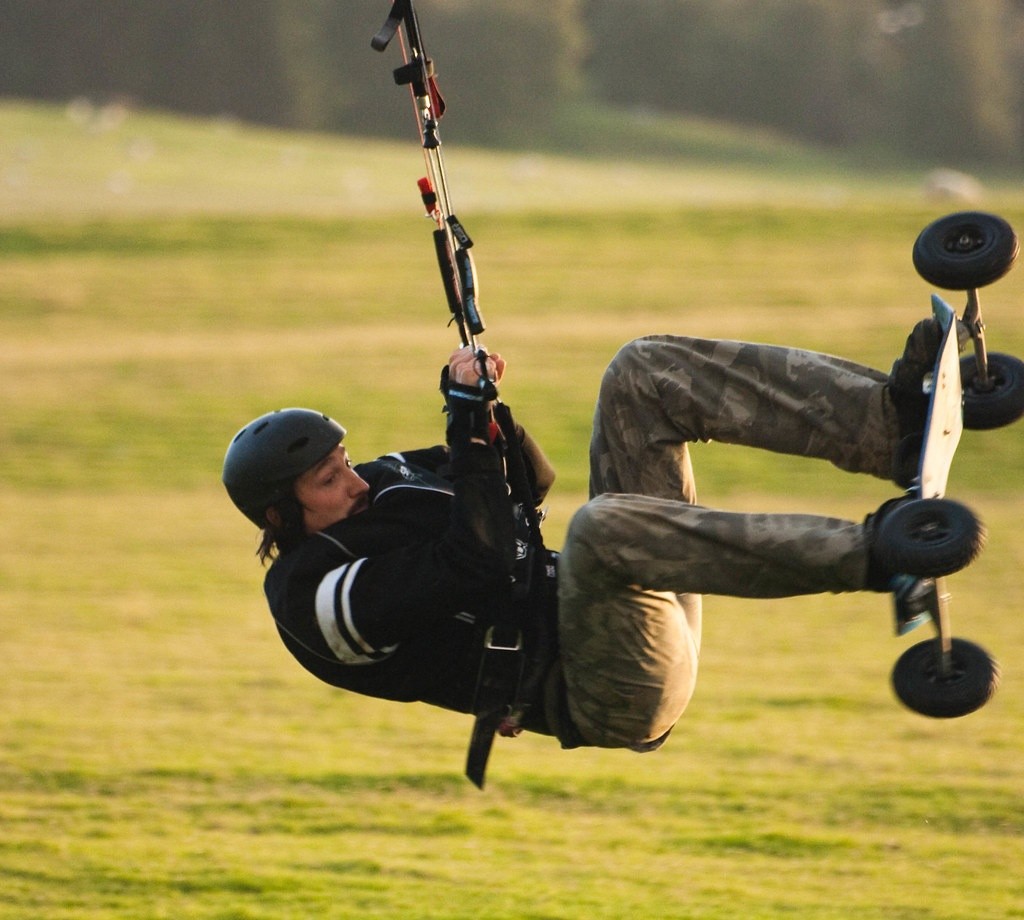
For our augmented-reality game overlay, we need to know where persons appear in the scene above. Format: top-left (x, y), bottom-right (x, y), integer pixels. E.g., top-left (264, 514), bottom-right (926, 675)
top-left (223, 319), bottom-right (942, 750)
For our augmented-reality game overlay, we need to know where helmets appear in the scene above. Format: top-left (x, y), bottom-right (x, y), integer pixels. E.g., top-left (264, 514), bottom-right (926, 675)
top-left (222, 408), bottom-right (347, 529)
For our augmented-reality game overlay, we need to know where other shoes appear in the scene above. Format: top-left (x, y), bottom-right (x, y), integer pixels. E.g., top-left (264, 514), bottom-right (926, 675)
top-left (887, 319), bottom-right (942, 489)
top-left (863, 491), bottom-right (921, 593)
